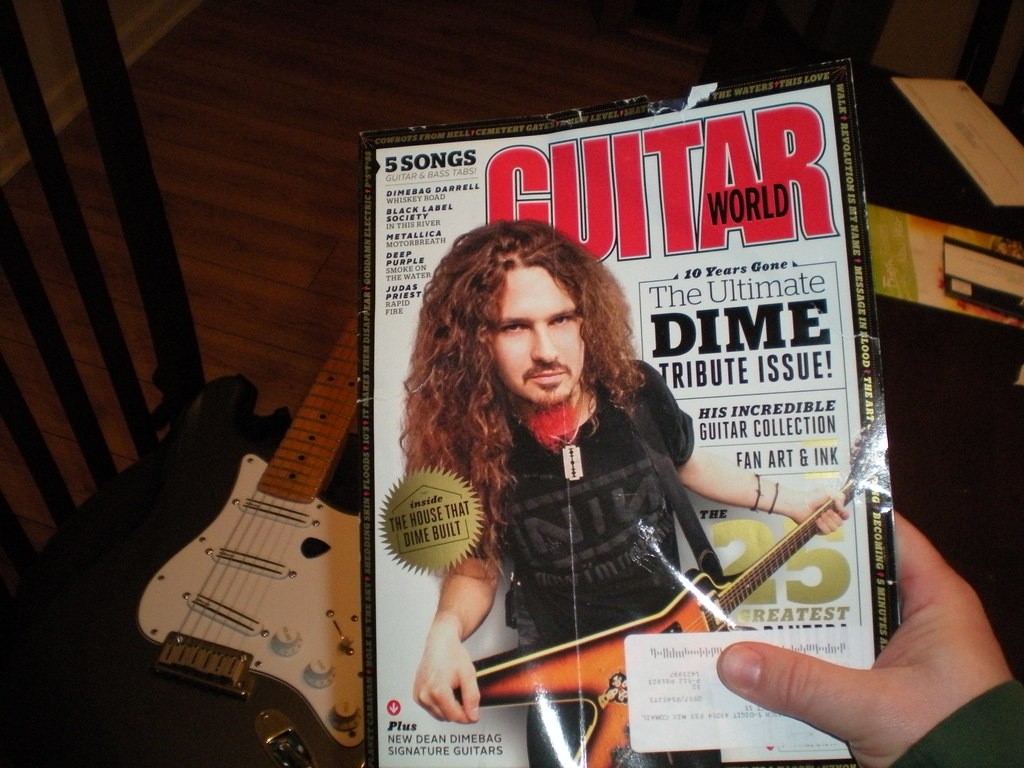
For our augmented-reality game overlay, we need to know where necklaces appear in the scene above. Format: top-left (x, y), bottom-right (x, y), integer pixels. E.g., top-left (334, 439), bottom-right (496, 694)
top-left (522, 382), bottom-right (585, 482)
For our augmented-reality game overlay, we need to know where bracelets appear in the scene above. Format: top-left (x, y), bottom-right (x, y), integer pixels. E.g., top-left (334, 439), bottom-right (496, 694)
top-left (769, 481), bottom-right (781, 512)
top-left (752, 471), bottom-right (763, 512)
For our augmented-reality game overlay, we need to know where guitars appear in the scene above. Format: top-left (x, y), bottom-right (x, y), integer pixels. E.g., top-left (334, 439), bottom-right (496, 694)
top-left (133, 302), bottom-right (360, 768)
top-left (451, 419), bottom-right (879, 768)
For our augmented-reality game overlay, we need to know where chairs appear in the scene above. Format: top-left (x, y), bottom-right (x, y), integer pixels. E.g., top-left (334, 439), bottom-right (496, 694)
top-left (0, 0), bottom-right (294, 768)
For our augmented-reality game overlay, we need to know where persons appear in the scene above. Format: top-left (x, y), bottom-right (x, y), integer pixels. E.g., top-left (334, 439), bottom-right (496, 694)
top-left (400, 218), bottom-right (849, 767)
top-left (717, 511), bottom-right (1024, 768)
